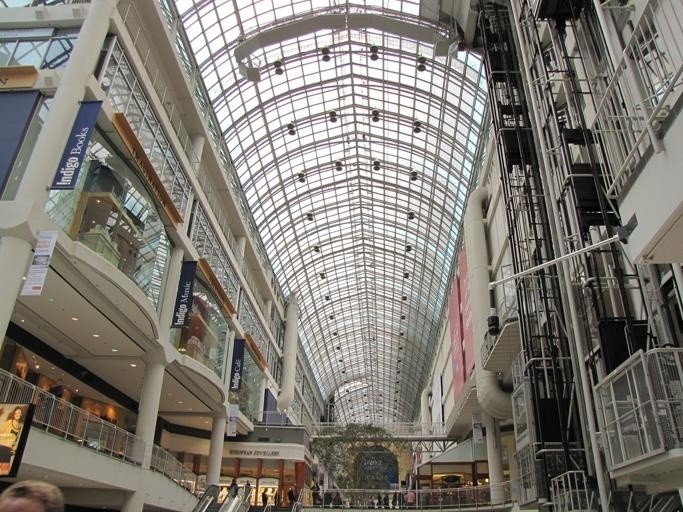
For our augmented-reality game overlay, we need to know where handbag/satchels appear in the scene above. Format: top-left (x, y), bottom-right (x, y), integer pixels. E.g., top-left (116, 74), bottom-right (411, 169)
top-left (230, 487), bottom-right (236, 496)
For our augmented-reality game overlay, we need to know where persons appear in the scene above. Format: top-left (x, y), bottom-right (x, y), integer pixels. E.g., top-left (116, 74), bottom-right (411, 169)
top-left (0, 407), bottom-right (23, 475)
top-left (0, 481), bottom-right (65, 512)
top-left (287, 487), bottom-right (296, 504)
top-left (244, 479), bottom-right (252, 503)
top-left (262, 488), bottom-right (268, 505)
top-left (310, 481), bottom-right (506, 510)
top-left (228, 480), bottom-right (239, 496)
